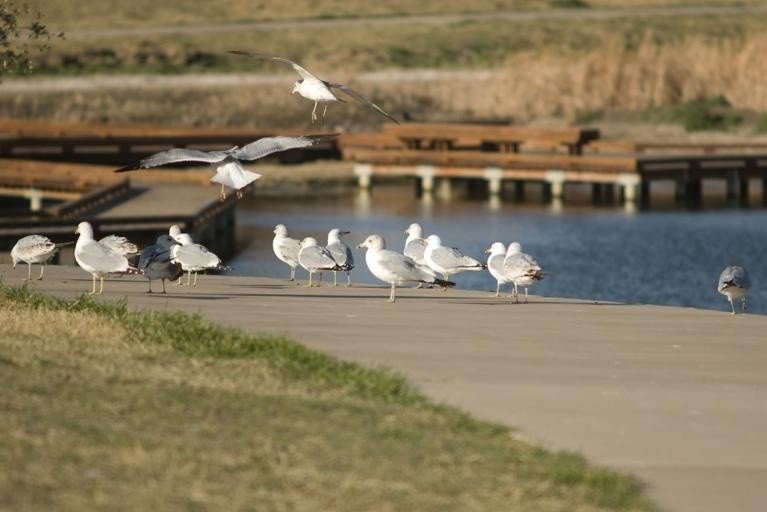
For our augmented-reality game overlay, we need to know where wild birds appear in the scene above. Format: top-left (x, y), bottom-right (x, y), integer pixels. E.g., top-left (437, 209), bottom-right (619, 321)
top-left (272, 223), bottom-right (303, 281)
top-left (356, 233), bottom-right (457, 303)
top-left (403, 222), bottom-right (552, 304)
top-left (112, 132), bottom-right (342, 202)
top-left (225, 50), bottom-right (400, 128)
top-left (324, 228), bottom-right (356, 287)
top-left (717, 264), bottom-right (752, 315)
top-left (10, 234), bottom-right (75, 281)
top-left (74, 221), bottom-right (139, 295)
top-left (297, 237), bottom-right (345, 288)
top-left (98, 225), bottom-right (232, 295)
